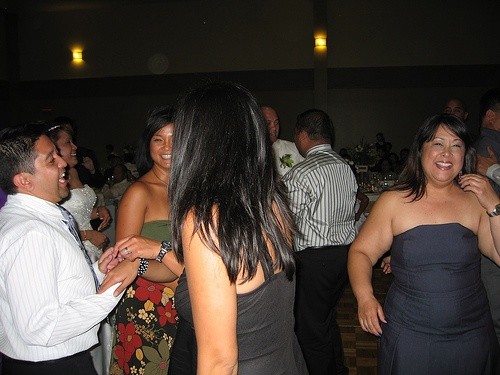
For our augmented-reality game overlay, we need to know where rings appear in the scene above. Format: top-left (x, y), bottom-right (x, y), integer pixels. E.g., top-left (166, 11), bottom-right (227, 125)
top-left (123, 246), bottom-right (130, 255)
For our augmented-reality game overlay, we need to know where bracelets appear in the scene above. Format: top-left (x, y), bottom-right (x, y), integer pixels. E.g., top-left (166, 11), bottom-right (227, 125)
top-left (83, 230), bottom-right (88, 242)
top-left (138, 258), bottom-right (150, 277)
top-left (96, 206), bottom-right (102, 220)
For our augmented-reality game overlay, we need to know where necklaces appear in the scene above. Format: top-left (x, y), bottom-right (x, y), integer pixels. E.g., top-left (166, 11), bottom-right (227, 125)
top-left (67, 175), bottom-right (71, 183)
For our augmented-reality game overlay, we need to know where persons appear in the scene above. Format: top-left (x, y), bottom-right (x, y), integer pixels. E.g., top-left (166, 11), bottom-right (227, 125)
top-left (0, 118), bottom-right (144, 375)
top-left (347, 87), bottom-right (500, 375)
top-left (108, 80), bottom-right (311, 375)
top-left (278, 109), bottom-right (368, 375)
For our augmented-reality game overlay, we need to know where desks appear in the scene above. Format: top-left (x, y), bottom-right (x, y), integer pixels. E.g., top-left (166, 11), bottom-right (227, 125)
top-left (355, 180), bottom-right (395, 218)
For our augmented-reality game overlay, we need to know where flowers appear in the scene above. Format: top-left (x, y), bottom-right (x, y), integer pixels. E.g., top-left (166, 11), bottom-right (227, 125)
top-left (278, 153), bottom-right (293, 168)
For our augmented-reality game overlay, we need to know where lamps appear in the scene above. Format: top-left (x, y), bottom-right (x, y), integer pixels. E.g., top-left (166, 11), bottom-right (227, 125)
top-left (313, 27), bottom-right (327, 57)
top-left (71, 46), bottom-right (84, 65)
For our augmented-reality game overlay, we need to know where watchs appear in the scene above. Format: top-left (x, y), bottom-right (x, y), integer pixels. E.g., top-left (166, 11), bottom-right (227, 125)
top-left (487, 203), bottom-right (500, 218)
top-left (156, 239), bottom-right (173, 262)
